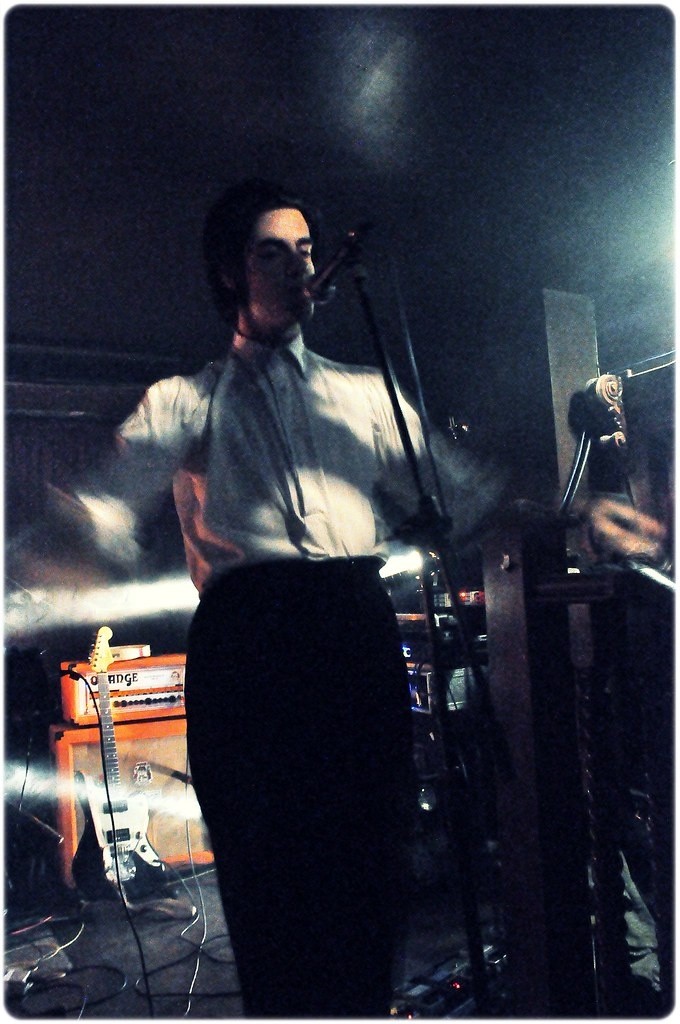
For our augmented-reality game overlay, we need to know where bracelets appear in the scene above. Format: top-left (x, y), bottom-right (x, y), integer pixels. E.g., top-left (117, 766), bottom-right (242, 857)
top-left (580, 495), bottom-right (602, 522)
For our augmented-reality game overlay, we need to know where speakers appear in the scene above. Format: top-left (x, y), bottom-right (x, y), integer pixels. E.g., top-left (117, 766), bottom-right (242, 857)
top-left (49, 719), bottom-right (216, 896)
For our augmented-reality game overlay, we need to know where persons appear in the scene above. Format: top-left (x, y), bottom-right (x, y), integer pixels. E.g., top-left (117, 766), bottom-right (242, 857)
top-left (13, 182), bottom-right (668, 1019)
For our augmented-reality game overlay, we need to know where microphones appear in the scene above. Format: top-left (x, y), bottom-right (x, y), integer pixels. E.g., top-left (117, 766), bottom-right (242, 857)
top-left (172, 771), bottom-right (193, 784)
top-left (303, 225), bottom-right (369, 305)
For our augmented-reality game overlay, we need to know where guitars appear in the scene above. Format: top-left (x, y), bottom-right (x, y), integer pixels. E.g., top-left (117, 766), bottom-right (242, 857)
top-left (70, 623), bottom-right (172, 904)
